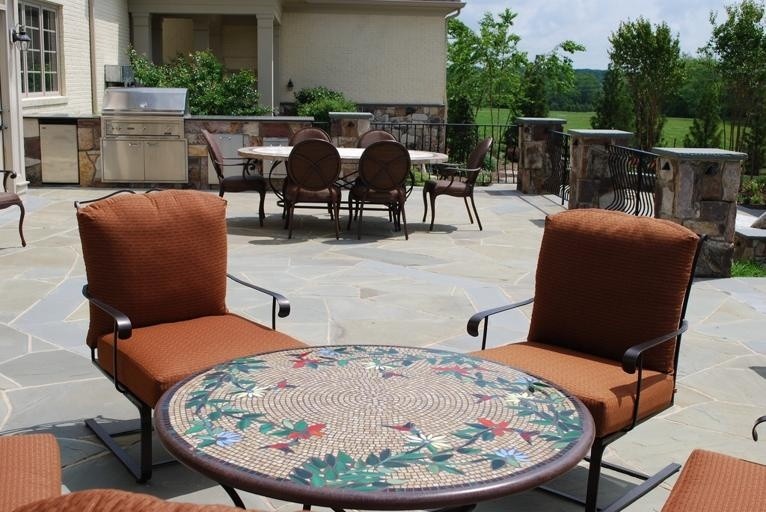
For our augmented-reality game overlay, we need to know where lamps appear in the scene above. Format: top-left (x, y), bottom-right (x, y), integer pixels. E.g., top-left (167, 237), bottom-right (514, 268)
top-left (286, 78), bottom-right (295, 91)
top-left (10, 21), bottom-right (32, 52)
